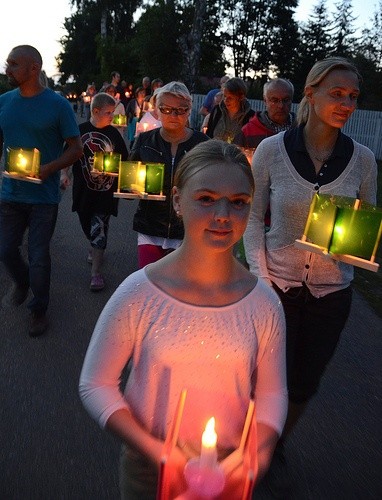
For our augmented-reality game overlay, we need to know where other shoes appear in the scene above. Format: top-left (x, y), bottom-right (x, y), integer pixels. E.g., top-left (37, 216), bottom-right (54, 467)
top-left (28, 313), bottom-right (47, 337)
top-left (11, 281), bottom-right (30, 305)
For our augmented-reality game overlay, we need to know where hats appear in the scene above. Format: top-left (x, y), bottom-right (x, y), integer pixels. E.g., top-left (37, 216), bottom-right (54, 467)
top-left (149, 87), bottom-right (160, 109)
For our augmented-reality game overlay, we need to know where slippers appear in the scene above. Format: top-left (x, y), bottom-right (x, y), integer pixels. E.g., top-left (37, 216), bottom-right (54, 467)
top-left (87, 252), bottom-right (94, 264)
top-left (90, 273), bottom-right (105, 290)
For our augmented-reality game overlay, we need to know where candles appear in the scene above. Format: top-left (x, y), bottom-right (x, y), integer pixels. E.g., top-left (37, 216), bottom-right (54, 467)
top-left (198, 418), bottom-right (217, 488)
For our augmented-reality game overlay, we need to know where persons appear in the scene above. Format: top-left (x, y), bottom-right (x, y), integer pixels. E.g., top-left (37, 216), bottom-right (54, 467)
top-left (122, 82), bottom-right (215, 280)
top-left (200, 75), bottom-right (231, 120)
top-left (58, 91), bottom-right (128, 290)
top-left (244, 56), bottom-right (379, 457)
top-left (79, 139), bottom-right (291, 499)
top-left (206, 76), bottom-right (256, 147)
top-left (60, 69), bottom-right (170, 157)
top-left (200, 92), bottom-right (224, 135)
top-left (0, 43), bottom-right (82, 337)
top-left (229, 77), bottom-right (300, 268)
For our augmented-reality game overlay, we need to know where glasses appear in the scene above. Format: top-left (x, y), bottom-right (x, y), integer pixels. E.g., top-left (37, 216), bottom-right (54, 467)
top-left (159, 106), bottom-right (190, 115)
